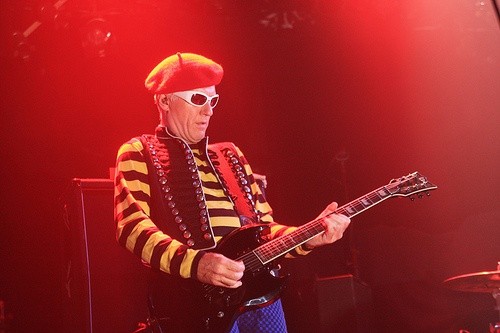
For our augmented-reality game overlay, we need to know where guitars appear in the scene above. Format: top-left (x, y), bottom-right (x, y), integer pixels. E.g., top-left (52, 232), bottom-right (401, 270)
top-left (146, 170), bottom-right (437, 333)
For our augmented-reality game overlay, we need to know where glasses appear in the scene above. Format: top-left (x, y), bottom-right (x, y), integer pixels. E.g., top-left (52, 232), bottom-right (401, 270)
top-left (173, 91), bottom-right (219, 108)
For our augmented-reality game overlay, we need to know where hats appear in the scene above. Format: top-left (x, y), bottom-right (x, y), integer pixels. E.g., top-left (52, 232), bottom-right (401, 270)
top-left (143, 52), bottom-right (224, 95)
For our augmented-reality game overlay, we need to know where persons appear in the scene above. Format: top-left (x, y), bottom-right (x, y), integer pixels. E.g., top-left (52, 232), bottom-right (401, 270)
top-left (113, 53), bottom-right (352, 333)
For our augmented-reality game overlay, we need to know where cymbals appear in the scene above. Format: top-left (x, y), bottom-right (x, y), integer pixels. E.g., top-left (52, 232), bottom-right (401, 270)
top-left (441, 269), bottom-right (499, 293)
